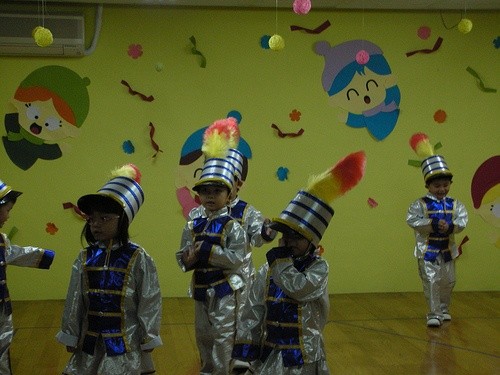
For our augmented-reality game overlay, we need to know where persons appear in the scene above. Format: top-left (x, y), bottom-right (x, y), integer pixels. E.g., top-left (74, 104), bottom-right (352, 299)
top-left (55, 176), bottom-right (164, 375)
top-left (0, 177), bottom-right (55, 375)
top-left (231, 191), bottom-right (334, 375)
top-left (176, 149), bottom-right (279, 375)
top-left (406, 154), bottom-right (469, 327)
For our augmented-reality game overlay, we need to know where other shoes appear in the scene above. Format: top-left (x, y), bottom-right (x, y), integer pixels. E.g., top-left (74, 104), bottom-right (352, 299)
top-left (442, 312), bottom-right (451, 319)
top-left (427, 318), bottom-right (440, 325)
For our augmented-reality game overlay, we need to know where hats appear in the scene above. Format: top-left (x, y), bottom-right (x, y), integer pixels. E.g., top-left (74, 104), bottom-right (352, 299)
top-left (77, 177), bottom-right (144, 228)
top-left (421, 155), bottom-right (453, 188)
top-left (226, 148), bottom-right (243, 181)
top-left (192, 158), bottom-right (235, 195)
top-left (0, 181), bottom-right (23, 205)
top-left (264, 190), bottom-right (335, 249)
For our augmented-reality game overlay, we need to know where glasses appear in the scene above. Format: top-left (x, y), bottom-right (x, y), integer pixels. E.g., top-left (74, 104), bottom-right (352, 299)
top-left (85, 215), bottom-right (120, 225)
